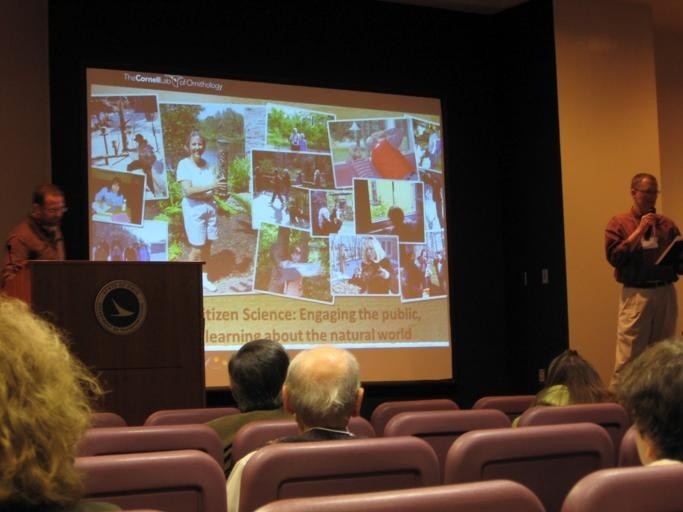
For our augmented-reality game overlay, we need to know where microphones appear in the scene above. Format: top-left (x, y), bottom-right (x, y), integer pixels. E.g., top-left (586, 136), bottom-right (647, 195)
top-left (8, 244), bottom-right (11, 264)
top-left (645, 206), bottom-right (656, 240)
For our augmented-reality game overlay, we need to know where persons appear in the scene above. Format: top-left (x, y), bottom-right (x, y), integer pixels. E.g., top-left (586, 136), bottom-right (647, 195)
top-left (401, 250), bottom-right (447, 298)
top-left (312, 191), bottom-right (347, 234)
top-left (603, 172), bottom-right (682, 390)
top-left (312, 169), bottom-right (328, 188)
top-left (0, 183), bottom-right (66, 295)
top-left (95, 177), bottom-right (124, 213)
top-left (122, 133), bottom-right (156, 195)
top-left (612, 334), bottom-right (682, 466)
top-left (289, 128), bottom-right (307, 152)
top-left (95, 236), bottom-right (151, 261)
top-left (253, 166), bottom-right (263, 192)
top-left (224, 343), bottom-right (365, 511)
top-left (176, 131), bottom-right (228, 293)
top-left (286, 200), bottom-right (304, 225)
top-left (199, 339), bottom-right (289, 444)
top-left (352, 235), bottom-right (399, 295)
top-left (268, 168), bottom-right (291, 206)
top-left (295, 170), bottom-right (302, 185)
top-left (0, 297), bottom-right (120, 511)
top-left (268, 229), bottom-right (308, 297)
top-left (510, 349), bottom-right (618, 428)
top-left (112, 140), bottom-right (119, 155)
top-left (347, 126), bottom-right (444, 241)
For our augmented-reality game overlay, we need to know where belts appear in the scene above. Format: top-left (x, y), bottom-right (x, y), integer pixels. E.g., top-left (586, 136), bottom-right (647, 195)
top-left (634, 281), bottom-right (673, 289)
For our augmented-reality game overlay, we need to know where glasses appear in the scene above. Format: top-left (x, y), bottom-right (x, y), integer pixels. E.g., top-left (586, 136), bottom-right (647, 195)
top-left (39, 206), bottom-right (67, 214)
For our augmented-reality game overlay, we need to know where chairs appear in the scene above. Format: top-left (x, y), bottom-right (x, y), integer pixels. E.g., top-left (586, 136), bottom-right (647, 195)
top-left (68, 390), bottom-right (681, 512)
